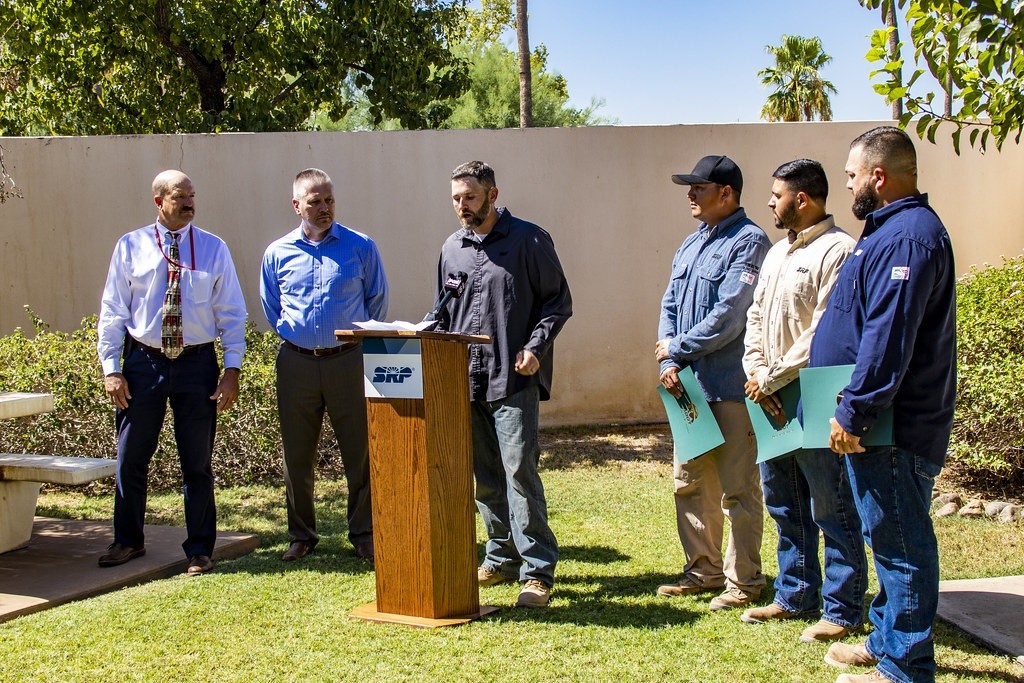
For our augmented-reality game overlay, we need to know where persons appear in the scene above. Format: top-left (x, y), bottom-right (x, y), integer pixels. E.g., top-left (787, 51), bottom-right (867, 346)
top-left (433, 160), bottom-right (575, 606)
top-left (741, 159), bottom-right (869, 645)
top-left (97, 169), bottom-right (247, 575)
top-left (259, 168), bottom-right (389, 565)
top-left (794, 126), bottom-right (956, 683)
top-left (655, 155), bottom-right (772, 612)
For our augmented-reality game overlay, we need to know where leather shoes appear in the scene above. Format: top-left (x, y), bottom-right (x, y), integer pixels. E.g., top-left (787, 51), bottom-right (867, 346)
top-left (187, 555), bottom-right (213, 576)
top-left (281, 542), bottom-right (313, 561)
top-left (97, 543), bottom-right (146, 567)
top-left (356, 538), bottom-right (374, 561)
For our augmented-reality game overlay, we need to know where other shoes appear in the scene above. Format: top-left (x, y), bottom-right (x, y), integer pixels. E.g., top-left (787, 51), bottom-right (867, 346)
top-left (743, 601), bottom-right (820, 622)
top-left (806, 616), bottom-right (865, 639)
top-left (710, 587), bottom-right (760, 610)
top-left (477, 566), bottom-right (519, 586)
top-left (824, 641), bottom-right (878, 667)
top-left (656, 576), bottom-right (709, 596)
top-left (518, 578), bottom-right (550, 606)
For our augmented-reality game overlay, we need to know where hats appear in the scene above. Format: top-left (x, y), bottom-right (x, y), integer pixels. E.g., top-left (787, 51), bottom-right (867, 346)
top-left (672, 155), bottom-right (744, 188)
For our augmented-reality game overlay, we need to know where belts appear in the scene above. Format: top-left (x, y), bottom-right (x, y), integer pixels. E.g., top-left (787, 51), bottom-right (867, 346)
top-left (284, 339), bottom-right (356, 356)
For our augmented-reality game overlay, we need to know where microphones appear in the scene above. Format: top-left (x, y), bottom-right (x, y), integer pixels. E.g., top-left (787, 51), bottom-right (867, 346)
top-left (421, 271), bottom-right (468, 331)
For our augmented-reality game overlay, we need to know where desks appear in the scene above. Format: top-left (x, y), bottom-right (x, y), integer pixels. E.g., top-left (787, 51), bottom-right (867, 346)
top-left (0, 392), bottom-right (53, 419)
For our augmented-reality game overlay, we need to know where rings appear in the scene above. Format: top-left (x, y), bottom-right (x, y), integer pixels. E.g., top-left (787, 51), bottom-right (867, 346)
top-left (232, 400), bottom-right (235, 404)
top-left (530, 368), bottom-right (534, 373)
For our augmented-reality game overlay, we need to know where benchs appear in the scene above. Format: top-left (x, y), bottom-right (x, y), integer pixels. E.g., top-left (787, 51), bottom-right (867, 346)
top-left (0, 451), bottom-right (119, 554)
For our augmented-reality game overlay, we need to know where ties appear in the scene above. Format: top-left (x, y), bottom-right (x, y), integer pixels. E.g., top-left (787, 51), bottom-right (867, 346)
top-left (161, 232), bottom-right (184, 360)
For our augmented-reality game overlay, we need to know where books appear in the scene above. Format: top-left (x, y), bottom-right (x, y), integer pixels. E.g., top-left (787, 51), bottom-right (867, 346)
top-left (744, 378), bottom-right (807, 465)
top-left (657, 365), bottom-right (726, 468)
top-left (799, 363), bottom-right (896, 450)
